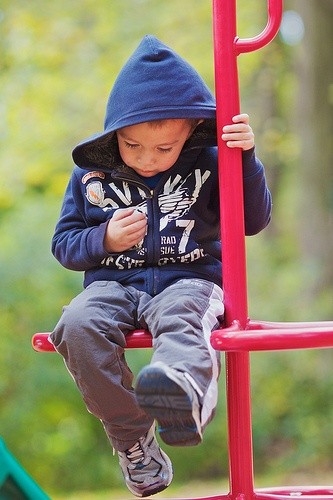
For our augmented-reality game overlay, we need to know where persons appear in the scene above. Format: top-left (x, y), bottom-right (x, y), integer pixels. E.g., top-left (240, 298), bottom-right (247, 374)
top-left (48, 34), bottom-right (274, 499)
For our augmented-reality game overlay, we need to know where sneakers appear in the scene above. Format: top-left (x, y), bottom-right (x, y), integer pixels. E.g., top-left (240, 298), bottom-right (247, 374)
top-left (113, 422), bottom-right (174, 498)
top-left (133, 361), bottom-right (207, 448)
